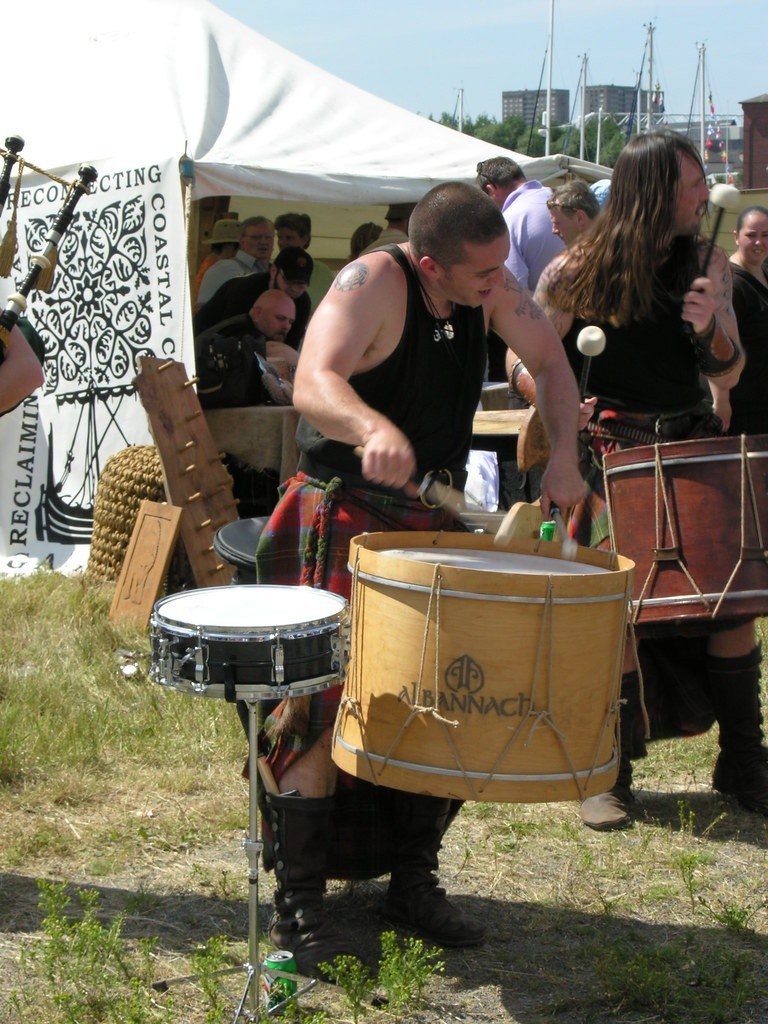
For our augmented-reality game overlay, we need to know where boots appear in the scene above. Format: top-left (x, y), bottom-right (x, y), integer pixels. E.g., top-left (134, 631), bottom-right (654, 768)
top-left (385, 795), bottom-right (476, 947)
top-left (581, 670), bottom-right (634, 827)
top-left (259, 790), bottom-right (369, 982)
top-left (702, 641), bottom-right (768, 817)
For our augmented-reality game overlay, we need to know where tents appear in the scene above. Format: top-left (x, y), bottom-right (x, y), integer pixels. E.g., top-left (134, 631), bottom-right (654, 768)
top-left (0, 0), bottom-right (620, 597)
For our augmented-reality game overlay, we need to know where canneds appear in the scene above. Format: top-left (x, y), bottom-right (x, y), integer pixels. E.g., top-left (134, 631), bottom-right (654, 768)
top-left (539, 520), bottom-right (557, 542)
top-left (261, 950), bottom-right (298, 1016)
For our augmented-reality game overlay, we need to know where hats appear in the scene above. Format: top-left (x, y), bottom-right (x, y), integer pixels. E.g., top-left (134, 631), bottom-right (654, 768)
top-left (200, 218), bottom-right (240, 245)
top-left (272, 246), bottom-right (314, 287)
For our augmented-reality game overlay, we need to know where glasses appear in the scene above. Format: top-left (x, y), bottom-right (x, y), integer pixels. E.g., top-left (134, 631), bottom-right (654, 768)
top-left (477, 161), bottom-right (498, 191)
top-left (547, 200), bottom-right (578, 212)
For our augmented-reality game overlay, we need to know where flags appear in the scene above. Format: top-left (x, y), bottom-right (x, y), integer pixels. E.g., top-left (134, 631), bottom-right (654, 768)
top-left (702, 92), bottom-right (734, 187)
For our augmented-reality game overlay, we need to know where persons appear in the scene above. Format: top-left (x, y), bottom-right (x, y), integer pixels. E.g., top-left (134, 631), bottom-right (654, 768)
top-left (507, 134), bottom-right (768, 831)
top-left (241, 180), bottom-right (587, 983)
top-left (193, 158), bottom-right (768, 519)
top-left (0, 318), bottom-right (44, 422)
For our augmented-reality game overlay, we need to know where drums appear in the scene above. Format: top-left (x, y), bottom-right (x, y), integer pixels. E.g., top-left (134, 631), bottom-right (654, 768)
top-left (602, 435), bottom-right (766, 620)
top-left (329, 533), bottom-right (642, 804)
top-left (149, 584), bottom-right (347, 700)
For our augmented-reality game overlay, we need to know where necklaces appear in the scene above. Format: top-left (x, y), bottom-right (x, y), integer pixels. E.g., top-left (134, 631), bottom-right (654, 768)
top-left (409, 252), bottom-right (456, 339)
top-left (423, 289), bottom-right (443, 341)
